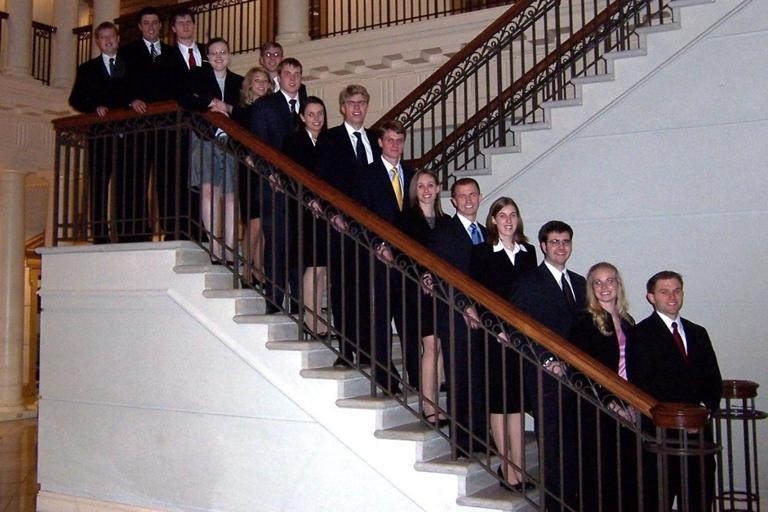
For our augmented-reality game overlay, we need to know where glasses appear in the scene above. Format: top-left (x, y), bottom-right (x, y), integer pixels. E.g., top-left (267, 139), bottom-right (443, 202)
top-left (545, 239), bottom-right (571, 247)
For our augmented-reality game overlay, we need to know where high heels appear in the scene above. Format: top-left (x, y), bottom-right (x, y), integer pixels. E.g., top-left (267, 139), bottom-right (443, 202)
top-left (302, 321), bottom-right (337, 339)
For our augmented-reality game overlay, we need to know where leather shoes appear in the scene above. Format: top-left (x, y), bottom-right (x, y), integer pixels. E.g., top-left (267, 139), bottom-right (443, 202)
top-left (209, 254), bottom-right (234, 267)
top-left (422, 407), bottom-right (448, 428)
top-left (241, 274), bottom-right (266, 289)
top-left (333, 351), bottom-right (354, 370)
top-left (383, 383), bottom-right (402, 399)
top-left (498, 464), bottom-right (536, 492)
top-left (409, 382), bottom-right (447, 392)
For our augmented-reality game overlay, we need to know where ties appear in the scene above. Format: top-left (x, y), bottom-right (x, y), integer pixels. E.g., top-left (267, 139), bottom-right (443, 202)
top-left (672, 322), bottom-right (686, 358)
top-left (289, 99), bottom-right (297, 113)
top-left (353, 131), bottom-right (368, 164)
top-left (150, 44), bottom-right (158, 59)
top-left (188, 48), bottom-right (196, 67)
top-left (470, 223), bottom-right (482, 244)
top-left (391, 167), bottom-right (403, 211)
top-left (561, 273), bottom-right (575, 302)
top-left (109, 58), bottom-right (115, 74)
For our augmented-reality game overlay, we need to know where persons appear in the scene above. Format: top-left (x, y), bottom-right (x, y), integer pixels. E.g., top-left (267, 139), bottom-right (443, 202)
top-left (279, 95), bottom-right (329, 341)
top-left (465, 196), bottom-right (538, 494)
top-left (428, 177), bottom-right (498, 462)
top-left (66, 21), bottom-right (126, 243)
top-left (571, 261), bottom-right (636, 512)
top-left (355, 117), bottom-right (423, 400)
top-left (112, 7), bottom-right (179, 240)
top-left (372, 168), bottom-right (451, 430)
top-left (162, 7), bottom-right (211, 242)
top-left (257, 39), bottom-right (283, 93)
top-left (622, 269), bottom-right (723, 512)
top-left (237, 67), bottom-right (276, 288)
top-left (239, 57), bottom-right (308, 318)
top-left (311, 83), bottom-right (385, 372)
top-left (512, 220), bottom-right (586, 512)
top-left (176, 37), bottom-right (242, 266)
